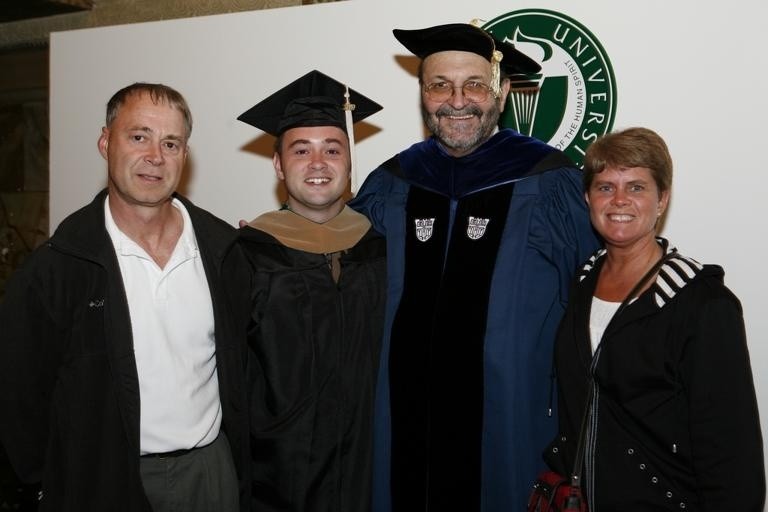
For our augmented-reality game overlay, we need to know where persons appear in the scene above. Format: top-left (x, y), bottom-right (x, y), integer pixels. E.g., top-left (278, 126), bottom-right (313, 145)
top-left (238, 28), bottom-right (598, 512)
top-left (1, 83), bottom-right (239, 512)
top-left (556, 127), bottom-right (763, 512)
top-left (216, 70), bottom-right (387, 512)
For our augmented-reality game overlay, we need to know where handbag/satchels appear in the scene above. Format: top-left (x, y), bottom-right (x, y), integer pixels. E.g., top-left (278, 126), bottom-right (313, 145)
top-left (528, 473), bottom-right (589, 512)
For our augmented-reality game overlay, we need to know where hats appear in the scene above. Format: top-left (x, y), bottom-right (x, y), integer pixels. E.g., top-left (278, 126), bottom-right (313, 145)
top-left (239, 69), bottom-right (384, 195)
top-left (391, 18), bottom-right (543, 99)
